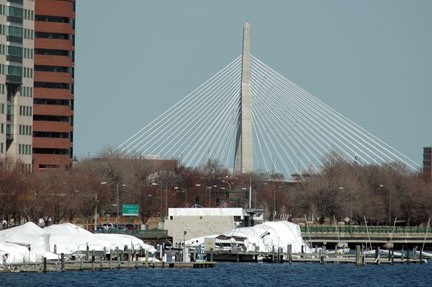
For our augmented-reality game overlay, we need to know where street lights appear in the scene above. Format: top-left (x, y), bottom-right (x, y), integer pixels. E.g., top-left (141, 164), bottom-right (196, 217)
top-left (93, 177), bottom-right (390, 231)
top-left (184, 231), bottom-right (187, 261)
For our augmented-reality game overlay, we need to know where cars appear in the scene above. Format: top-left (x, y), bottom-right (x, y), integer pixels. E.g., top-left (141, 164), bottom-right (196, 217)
top-left (109, 225), bottom-right (128, 234)
top-left (99, 223), bottom-right (117, 233)
